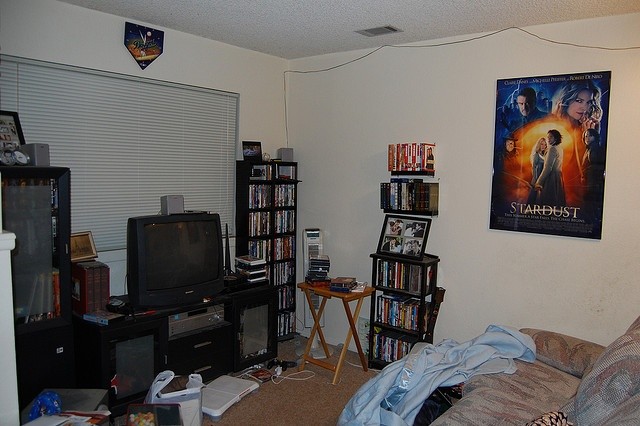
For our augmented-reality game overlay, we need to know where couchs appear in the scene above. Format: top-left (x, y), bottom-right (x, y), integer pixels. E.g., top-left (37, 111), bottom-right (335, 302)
top-left (429, 317), bottom-right (637, 425)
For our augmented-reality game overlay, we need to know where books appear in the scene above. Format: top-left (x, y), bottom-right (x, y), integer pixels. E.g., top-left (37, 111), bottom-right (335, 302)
top-left (249, 212), bottom-right (271, 237)
top-left (274, 237), bottom-right (295, 260)
top-left (275, 164), bottom-right (295, 180)
top-left (305, 255), bottom-right (331, 287)
top-left (330, 277), bottom-right (354, 292)
top-left (278, 311), bottom-right (296, 336)
top-left (378, 260), bottom-right (430, 294)
top-left (372, 327), bottom-right (412, 364)
top-left (15, 266), bottom-right (61, 323)
top-left (4, 217), bottom-right (58, 253)
top-left (249, 240), bottom-right (270, 261)
top-left (71, 262), bottom-right (110, 318)
top-left (387, 143), bottom-right (438, 172)
top-left (275, 210), bottom-right (294, 233)
top-left (279, 287), bottom-right (294, 309)
top-left (277, 148), bottom-right (294, 162)
top-left (274, 262), bottom-right (294, 286)
top-left (375, 290), bottom-right (431, 333)
top-left (379, 181), bottom-right (439, 211)
top-left (2, 175), bottom-right (58, 213)
top-left (249, 184), bottom-right (271, 208)
top-left (274, 184), bottom-right (295, 207)
top-left (265, 265), bottom-right (271, 285)
top-left (236, 255), bottom-right (267, 284)
top-left (250, 165), bottom-right (272, 181)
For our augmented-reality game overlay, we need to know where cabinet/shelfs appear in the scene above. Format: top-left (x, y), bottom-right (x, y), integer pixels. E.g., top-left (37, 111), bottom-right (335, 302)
top-left (0, 231), bottom-right (21, 425)
top-left (368, 252), bottom-right (440, 370)
top-left (72, 273), bottom-right (279, 418)
top-left (0, 167), bottom-right (72, 411)
top-left (236, 159), bottom-right (302, 341)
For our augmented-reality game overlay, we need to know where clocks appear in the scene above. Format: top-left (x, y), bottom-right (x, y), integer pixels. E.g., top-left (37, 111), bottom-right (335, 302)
top-left (124, 22), bottom-right (164, 71)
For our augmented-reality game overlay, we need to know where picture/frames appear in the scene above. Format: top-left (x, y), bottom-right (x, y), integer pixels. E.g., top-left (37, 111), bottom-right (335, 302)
top-left (242, 141), bottom-right (262, 162)
top-left (70, 230), bottom-right (99, 263)
top-left (0, 110), bottom-right (26, 149)
top-left (376, 214), bottom-right (432, 261)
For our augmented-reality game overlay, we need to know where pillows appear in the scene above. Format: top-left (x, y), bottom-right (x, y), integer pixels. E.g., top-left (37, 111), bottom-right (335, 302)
top-left (528, 411), bottom-right (571, 425)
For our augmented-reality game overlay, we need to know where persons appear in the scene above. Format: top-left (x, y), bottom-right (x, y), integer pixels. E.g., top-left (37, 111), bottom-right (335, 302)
top-left (509, 87), bottom-right (548, 131)
top-left (582, 128), bottom-right (604, 189)
top-left (530, 137), bottom-right (547, 207)
top-left (384, 217), bottom-right (423, 257)
top-left (496, 132), bottom-right (520, 164)
top-left (552, 80), bottom-right (603, 134)
top-left (534, 130), bottom-right (567, 207)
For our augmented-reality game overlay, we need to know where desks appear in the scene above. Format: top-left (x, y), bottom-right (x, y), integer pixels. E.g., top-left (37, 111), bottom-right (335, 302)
top-left (298, 279), bottom-right (375, 386)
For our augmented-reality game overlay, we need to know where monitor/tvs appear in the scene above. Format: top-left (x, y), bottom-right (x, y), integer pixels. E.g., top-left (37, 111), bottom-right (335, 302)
top-left (127, 212), bottom-right (226, 314)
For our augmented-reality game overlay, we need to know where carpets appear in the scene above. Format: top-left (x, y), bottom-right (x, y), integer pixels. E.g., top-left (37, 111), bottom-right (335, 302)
top-left (199, 347), bottom-right (382, 426)
